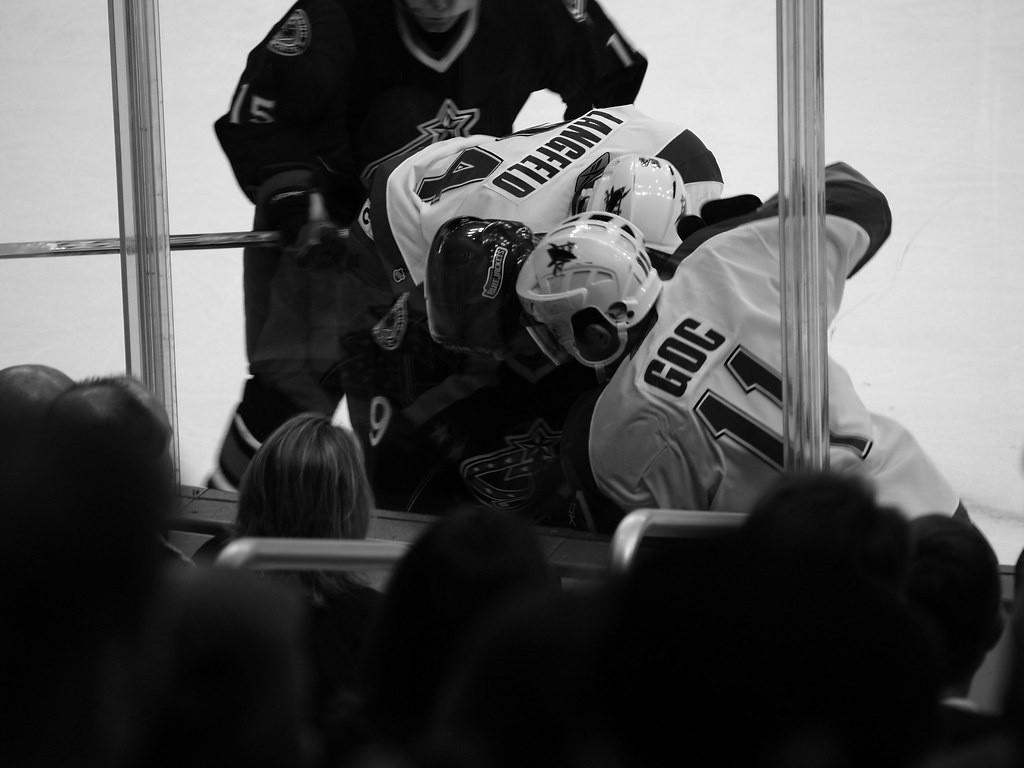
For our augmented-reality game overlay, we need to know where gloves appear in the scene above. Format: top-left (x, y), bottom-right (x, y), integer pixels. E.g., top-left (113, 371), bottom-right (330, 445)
top-left (252, 169), bottom-right (341, 259)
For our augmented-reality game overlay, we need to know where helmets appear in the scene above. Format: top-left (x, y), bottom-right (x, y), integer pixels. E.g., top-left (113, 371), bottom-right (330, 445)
top-left (425, 215), bottom-right (546, 372)
top-left (519, 209), bottom-right (663, 370)
top-left (571, 151), bottom-right (687, 254)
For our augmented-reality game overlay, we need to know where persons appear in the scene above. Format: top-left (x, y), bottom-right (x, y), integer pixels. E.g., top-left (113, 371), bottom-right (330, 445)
top-left (189, 411), bottom-right (377, 572)
top-left (0, 0), bottom-right (1024, 768)
